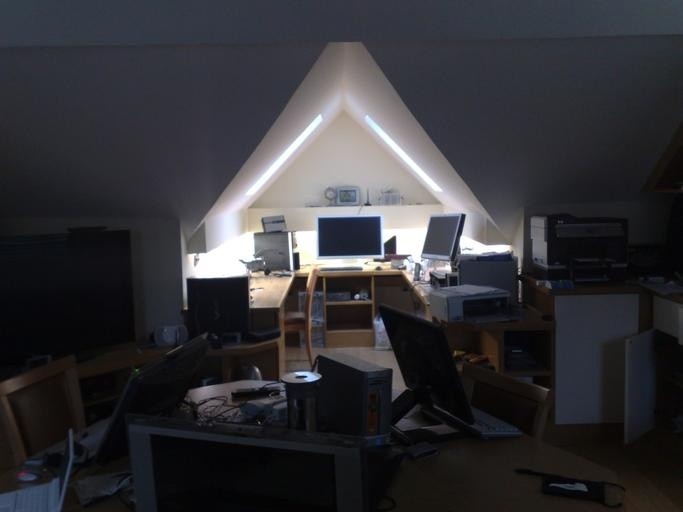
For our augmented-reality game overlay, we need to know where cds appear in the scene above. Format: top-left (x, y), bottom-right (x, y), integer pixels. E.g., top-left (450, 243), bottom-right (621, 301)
top-left (280, 369), bottom-right (323, 385)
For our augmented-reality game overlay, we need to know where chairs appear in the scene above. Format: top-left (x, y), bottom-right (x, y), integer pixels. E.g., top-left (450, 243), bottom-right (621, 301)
top-left (284, 264), bottom-right (320, 367)
top-left (0, 355), bottom-right (86, 464)
top-left (470, 369), bottom-right (552, 439)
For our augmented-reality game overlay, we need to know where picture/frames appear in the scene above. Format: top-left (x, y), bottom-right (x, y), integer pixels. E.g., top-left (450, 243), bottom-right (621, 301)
top-left (336, 186), bottom-right (360, 206)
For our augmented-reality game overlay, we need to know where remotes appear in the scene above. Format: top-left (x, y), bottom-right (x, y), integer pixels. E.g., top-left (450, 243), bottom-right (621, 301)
top-left (230, 385), bottom-right (282, 399)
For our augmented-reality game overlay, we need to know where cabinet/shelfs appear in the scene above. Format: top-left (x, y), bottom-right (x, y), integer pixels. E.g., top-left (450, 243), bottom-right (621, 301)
top-left (518, 275), bottom-right (651, 431)
top-left (623, 295), bottom-right (683, 445)
top-left (482, 304), bottom-right (554, 417)
top-left (323, 271), bottom-right (375, 347)
top-left (77, 339), bottom-right (286, 426)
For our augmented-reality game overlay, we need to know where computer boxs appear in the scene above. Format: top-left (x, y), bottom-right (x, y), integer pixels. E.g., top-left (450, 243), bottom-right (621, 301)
top-left (253, 231), bottom-right (294, 272)
top-left (457, 255), bottom-right (519, 309)
top-left (316, 351), bottom-right (393, 436)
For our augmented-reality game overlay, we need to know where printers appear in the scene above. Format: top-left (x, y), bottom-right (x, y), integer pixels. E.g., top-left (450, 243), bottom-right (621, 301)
top-left (529, 210), bottom-right (632, 286)
top-left (429, 284), bottom-right (512, 325)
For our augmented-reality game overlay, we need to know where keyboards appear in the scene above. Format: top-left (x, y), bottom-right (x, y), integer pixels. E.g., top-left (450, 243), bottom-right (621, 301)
top-left (471, 401), bottom-right (524, 441)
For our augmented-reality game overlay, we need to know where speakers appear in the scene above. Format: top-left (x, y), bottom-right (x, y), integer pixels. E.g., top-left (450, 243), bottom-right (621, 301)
top-left (429, 270), bottom-right (446, 289)
top-left (293, 251), bottom-right (301, 271)
top-left (447, 273), bottom-right (458, 286)
top-left (413, 262), bottom-right (422, 284)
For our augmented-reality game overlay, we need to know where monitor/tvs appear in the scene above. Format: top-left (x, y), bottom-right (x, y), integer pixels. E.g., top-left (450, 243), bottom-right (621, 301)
top-left (376, 300), bottom-right (479, 430)
top-left (318, 216), bottom-right (381, 270)
top-left (420, 212), bottom-right (466, 272)
top-left (95, 330), bottom-right (209, 468)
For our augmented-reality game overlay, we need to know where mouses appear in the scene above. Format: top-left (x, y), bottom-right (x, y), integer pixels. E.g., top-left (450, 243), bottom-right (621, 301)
top-left (375, 265), bottom-right (383, 271)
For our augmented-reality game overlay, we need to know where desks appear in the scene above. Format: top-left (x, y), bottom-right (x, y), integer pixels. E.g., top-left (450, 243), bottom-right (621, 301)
top-left (402, 269), bottom-right (431, 321)
top-left (249, 273), bottom-right (295, 339)
top-left (0, 380), bottom-right (625, 512)
